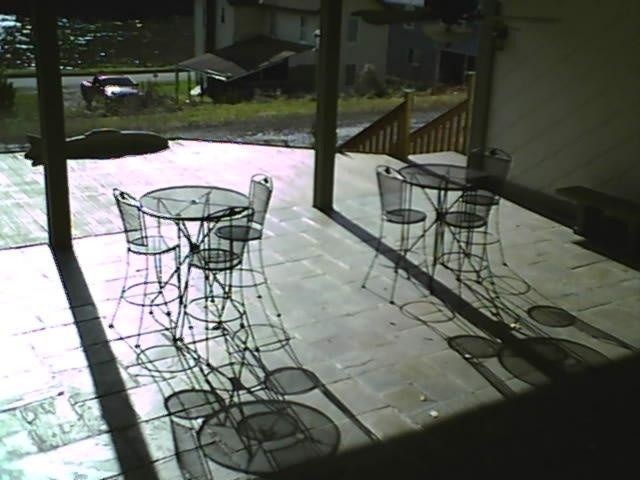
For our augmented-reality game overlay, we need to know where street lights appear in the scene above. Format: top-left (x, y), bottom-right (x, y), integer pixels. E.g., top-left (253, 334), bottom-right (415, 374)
top-left (311, 28), bottom-right (320, 101)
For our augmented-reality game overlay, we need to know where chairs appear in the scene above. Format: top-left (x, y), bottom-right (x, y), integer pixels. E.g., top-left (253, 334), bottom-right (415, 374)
top-left (179, 205), bottom-right (261, 350)
top-left (205, 172), bottom-right (281, 318)
top-left (112, 187), bottom-right (188, 348)
top-left (430, 177), bottom-right (494, 286)
top-left (361, 165), bottom-right (431, 305)
top-left (447, 148), bottom-right (512, 267)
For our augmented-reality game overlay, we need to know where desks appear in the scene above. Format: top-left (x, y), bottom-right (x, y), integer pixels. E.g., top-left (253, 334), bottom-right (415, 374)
top-left (140, 185), bottom-right (255, 328)
top-left (393, 163), bottom-right (488, 273)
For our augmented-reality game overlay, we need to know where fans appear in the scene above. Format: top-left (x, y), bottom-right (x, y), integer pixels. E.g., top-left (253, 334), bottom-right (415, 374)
top-left (349, 0), bottom-right (558, 37)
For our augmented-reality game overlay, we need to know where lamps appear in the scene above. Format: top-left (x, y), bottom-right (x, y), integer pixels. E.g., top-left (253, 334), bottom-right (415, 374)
top-left (430, 27), bottom-right (467, 48)
top-left (489, 22), bottom-right (509, 50)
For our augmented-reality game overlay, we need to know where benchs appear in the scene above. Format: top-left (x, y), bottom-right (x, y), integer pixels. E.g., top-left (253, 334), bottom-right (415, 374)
top-left (551, 183), bottom-right (639, 260)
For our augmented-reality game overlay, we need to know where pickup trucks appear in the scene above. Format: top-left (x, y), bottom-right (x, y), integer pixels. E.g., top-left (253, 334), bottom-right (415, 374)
top-left (80, 74), bottom-right (144, 112)
